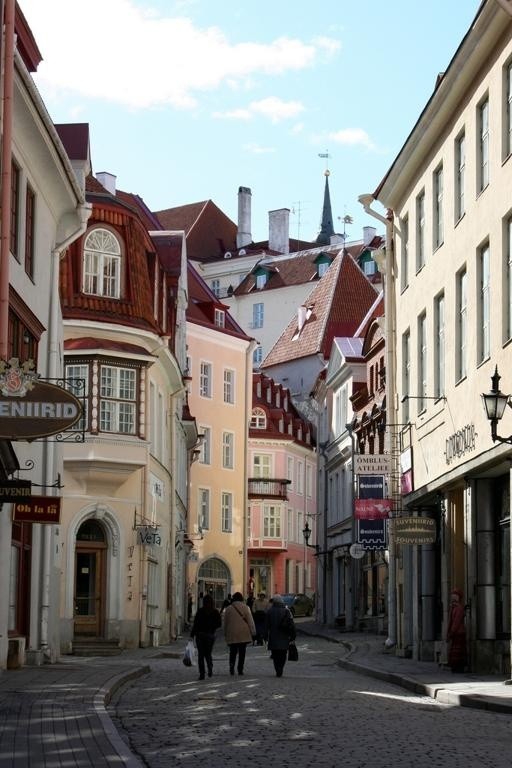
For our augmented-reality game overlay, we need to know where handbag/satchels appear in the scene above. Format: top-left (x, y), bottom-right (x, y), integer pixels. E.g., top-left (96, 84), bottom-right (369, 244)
top-left (287, 643), bottom-right (299, 661)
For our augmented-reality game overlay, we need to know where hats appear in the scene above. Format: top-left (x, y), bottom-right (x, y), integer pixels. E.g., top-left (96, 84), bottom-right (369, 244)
top-left (271, 593), bottom-right (284, 603)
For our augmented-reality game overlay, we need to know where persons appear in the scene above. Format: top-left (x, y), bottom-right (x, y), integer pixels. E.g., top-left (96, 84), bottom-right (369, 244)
top-left (187, 588), bottom-right (297, 681)
top-left (247, 576), bottom-right (255, 597)
top-left (446, 590), bottom-right (467, 674)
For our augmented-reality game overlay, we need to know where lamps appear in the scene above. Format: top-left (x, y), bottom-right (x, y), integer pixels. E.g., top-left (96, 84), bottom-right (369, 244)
top-left (303, 522), bottom-right (316, 549)
top-left (479, 364), bottom-right (512, 444)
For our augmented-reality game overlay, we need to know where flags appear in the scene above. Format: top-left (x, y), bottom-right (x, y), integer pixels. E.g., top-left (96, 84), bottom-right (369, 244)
top-left (356, 474), bottom-right (389, 553)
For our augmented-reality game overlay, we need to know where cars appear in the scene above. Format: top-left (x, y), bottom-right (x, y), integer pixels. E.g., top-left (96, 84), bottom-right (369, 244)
top-left (281, 593), bottom-right (314, 618)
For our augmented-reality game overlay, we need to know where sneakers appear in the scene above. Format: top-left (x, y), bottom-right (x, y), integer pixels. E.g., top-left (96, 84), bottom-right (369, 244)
top-left (229, 663), bottom-right (244, 676)
top-left (197, 666), bottom-right (213, 680)
